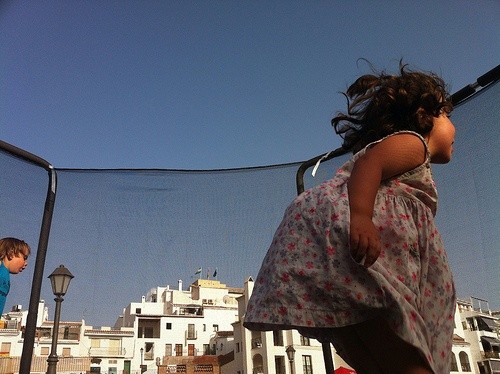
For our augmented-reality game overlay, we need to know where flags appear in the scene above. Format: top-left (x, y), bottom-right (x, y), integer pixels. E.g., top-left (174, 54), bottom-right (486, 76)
top-left (195, 266), bottom-right (218, 278)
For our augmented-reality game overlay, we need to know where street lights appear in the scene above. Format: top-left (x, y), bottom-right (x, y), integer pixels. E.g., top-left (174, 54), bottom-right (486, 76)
top-left (46, 264), bottom-right (75, 374)
top-left (285, 345), bottom-right (296, 374)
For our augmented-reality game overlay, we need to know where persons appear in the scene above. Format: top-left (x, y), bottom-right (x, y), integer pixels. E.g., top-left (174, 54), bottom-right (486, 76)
top-left (0, 237), bottom-right (32, 317)
top-left (243, 57), bottom-right (457, 374)
